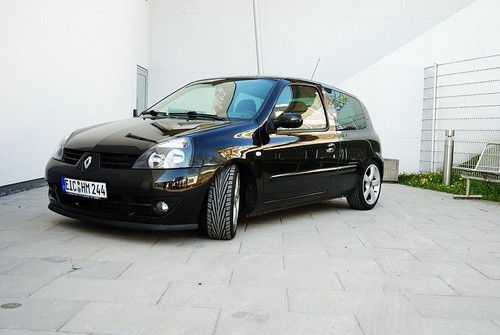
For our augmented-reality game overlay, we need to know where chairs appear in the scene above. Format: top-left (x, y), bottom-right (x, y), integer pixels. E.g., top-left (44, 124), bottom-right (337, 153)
top-left (235, 99), bottom-right (256, 113)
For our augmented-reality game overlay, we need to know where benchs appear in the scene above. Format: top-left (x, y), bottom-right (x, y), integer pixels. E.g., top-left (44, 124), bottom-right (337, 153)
top-left (452, 142), bottom-right (500, 199)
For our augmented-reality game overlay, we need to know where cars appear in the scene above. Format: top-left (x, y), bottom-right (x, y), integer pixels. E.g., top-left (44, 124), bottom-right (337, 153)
top-left (44, 58), bottom-right (384, 240)
